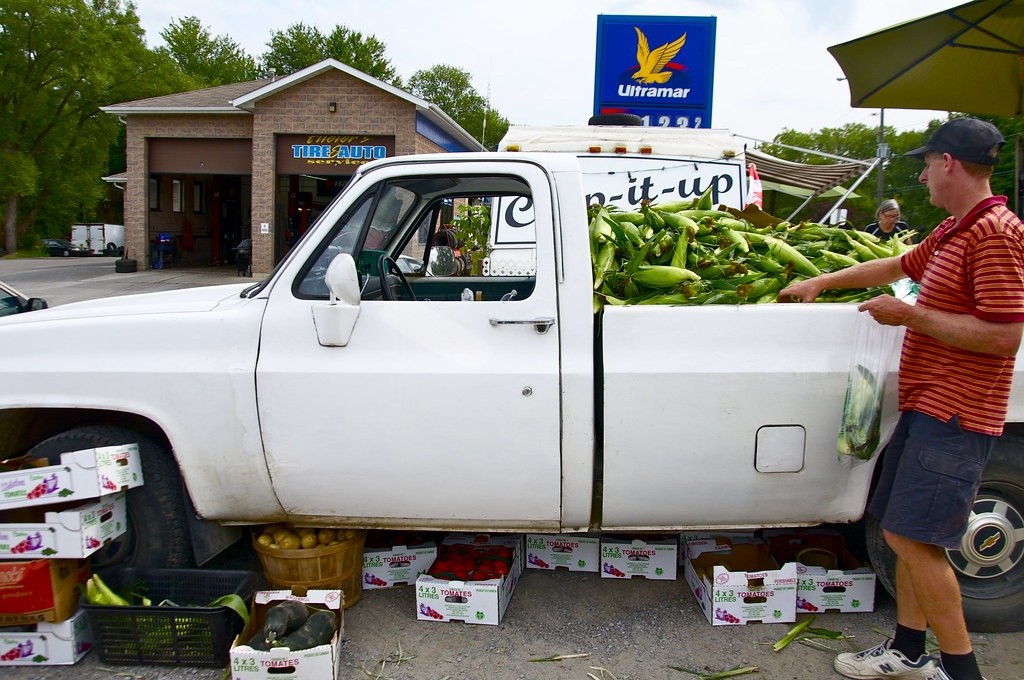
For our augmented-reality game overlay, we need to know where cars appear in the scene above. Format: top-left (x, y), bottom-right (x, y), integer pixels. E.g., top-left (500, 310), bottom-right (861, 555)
top-left (395, 255), bottom-right (434, 277)
top-left (0, 283), bottom-right (47, 316)
top-left (42, 239), bottom-right (95, 257)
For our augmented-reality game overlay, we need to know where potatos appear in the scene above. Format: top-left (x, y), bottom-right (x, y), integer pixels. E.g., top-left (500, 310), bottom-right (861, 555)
top-left (258, 528), bottom-right (360, 549)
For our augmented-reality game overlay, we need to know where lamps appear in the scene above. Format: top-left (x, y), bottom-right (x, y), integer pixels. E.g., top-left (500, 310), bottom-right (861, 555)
top-left (328, 101), bottom-right (337, 112)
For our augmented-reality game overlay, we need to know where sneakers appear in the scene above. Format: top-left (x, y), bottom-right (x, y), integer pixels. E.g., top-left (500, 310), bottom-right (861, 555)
top-left (923, 655), bottom-right (988, 680)
top-left (833, 637), bottom-right (936, 680)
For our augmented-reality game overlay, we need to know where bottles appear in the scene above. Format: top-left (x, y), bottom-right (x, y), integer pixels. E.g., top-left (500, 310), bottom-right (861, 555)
top-left (461, 288), bottom-right (474, 301)
top-left (499, 289), bottom-right (517, 301)
top-left (159, 232), bottom-right (170, 242)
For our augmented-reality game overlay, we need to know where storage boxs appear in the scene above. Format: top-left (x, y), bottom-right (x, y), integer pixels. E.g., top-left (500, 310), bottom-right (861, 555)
top-left (0, 557), bottom-right (89, 626)
top-left (229, 588), bottom-right (346, 680)
top-left (0, 608), bottom-right (95, 666)
top-left (0, 442), bottom-right (145, 511)
top-left (362, 538), bottom-right (438, 592)
top-left (525, 532), bottom-right (600, 573)
top-left (415, 539), bottom-right (520, 626)
top-left (772, 537), bottom-right (876, 613)
top-left (684, 538), bottom-right (797, 626)
top-left (679, 529), bottom-right (754, 566)
top-left (601, 536), bottom-right (679, 582)
top-left (0, 492), bottom-right (127, 560)
top-left (78, 566), bottom-right (255, 667)
top-left (443, 532), bottom-right (525, 573)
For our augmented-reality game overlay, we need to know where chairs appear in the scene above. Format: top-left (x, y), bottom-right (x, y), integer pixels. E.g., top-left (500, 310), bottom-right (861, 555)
top-left (231, 246), bottom-right (251, 277)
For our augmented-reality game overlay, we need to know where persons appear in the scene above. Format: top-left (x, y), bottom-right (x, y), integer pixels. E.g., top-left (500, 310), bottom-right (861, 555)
top-left (865, 200), bottom-right (911, 244)
top-left (780, 116), bottom-right (1024, 680)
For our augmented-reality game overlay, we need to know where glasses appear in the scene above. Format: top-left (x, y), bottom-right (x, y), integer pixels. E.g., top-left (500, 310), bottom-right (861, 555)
top-left (882, 212), bottom-right (900, 219)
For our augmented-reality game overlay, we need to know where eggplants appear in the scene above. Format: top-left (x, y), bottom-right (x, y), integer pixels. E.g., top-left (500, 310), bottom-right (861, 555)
top-left (249, 600), bottom-right (338, 649)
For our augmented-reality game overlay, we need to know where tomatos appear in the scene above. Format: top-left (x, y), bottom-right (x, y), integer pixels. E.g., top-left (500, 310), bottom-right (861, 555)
top-left (432, 545), bottom-right (511, 581)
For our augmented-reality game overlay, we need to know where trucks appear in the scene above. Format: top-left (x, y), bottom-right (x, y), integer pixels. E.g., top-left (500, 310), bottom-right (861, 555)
top-left (72, 222), bottom-right (124, 255)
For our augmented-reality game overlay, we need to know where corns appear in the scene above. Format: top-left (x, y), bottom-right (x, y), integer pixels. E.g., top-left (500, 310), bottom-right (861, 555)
top-left (588, 190), bottom-right (907, 313)
top-left (86, 574), bottom-right (211, 655)
top-left (838, 387), bottom-right (882, 460)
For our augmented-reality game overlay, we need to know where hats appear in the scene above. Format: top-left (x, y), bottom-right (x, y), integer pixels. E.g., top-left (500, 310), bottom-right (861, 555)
top-left (901, 115), bottom-right (1008, 165)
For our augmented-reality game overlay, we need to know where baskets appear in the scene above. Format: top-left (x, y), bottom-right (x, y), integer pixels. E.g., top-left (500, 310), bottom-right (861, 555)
top-left (76, 568), bottom-right (258, 667)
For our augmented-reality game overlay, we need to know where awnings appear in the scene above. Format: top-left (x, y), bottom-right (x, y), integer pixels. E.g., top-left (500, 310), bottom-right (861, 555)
top-left (733, 133), bottom-right (882, 225)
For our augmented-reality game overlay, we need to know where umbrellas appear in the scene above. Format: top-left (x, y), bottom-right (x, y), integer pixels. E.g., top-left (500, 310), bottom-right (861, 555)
top-left (827, 0), bottom-right (1024, 117)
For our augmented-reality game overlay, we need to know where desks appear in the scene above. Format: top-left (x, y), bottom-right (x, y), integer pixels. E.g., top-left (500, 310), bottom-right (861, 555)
top-left (150, 244), bottom-right (176, 270)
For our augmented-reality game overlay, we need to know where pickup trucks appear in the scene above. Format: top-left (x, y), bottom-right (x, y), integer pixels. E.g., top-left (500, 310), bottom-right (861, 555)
top-left (0, 150), bottom-right (1022, 636)
